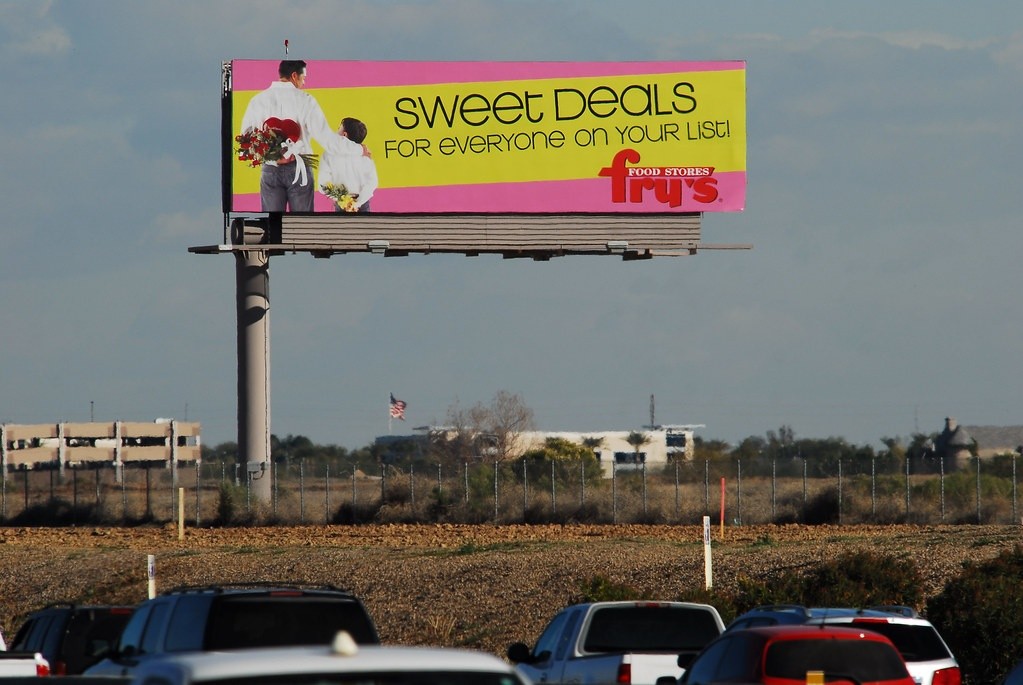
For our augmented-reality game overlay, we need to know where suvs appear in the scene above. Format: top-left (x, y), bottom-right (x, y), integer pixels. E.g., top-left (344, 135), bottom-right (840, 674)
top-left (6, 601), bottom-right (136, 678)
top-left (720, 602), bottom-right (960, 685)
top-left (78, 579), bottom-right (381, 685)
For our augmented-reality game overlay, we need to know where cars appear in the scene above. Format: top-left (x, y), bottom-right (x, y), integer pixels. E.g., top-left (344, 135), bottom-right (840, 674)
top-left (652, 623), bottom-right (915, 685)
top-left (122, 631), bottom-right (532, 685)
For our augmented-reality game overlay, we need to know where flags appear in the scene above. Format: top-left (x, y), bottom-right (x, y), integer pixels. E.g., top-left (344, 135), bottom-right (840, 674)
top-left (389, 392), bottom-right (407, 420)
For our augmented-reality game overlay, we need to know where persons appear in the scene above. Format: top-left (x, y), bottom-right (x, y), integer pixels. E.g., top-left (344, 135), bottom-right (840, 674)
top-left (240, 60), bottom-right (371, 213)
top-left (316, 117), bottom-right (376, 212)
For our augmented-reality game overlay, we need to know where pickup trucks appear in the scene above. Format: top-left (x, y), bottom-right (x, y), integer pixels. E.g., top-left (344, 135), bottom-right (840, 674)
top-left (505, 600), bottom-right (725, 685)
top-left (0, 634), bottom-right (51, 685)
